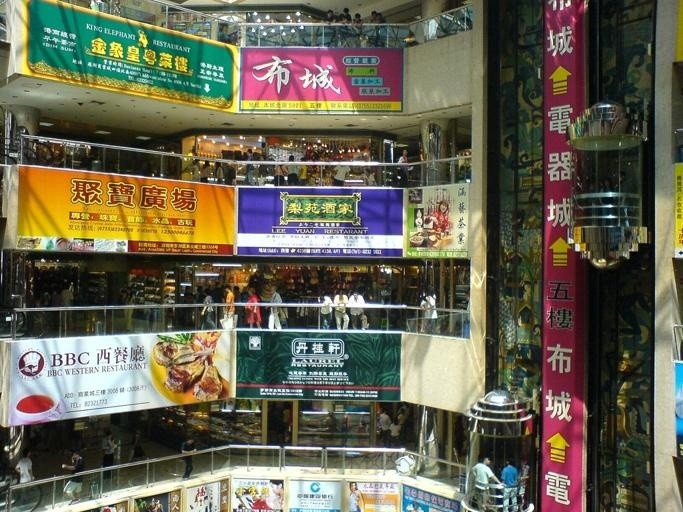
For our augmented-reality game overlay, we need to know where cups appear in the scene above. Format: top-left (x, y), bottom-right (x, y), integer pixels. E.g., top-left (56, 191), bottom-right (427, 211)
top-left (14, 394), bottom-right (60, 421)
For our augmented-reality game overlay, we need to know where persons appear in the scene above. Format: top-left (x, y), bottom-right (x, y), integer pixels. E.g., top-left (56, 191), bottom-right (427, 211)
top-left (85, 1), bottom-right (473, 47)
top-left (458, 437), bottom-right (470, 465)
top-left (181, 435), bottom-right (197, 480)
top-left (270, 479), bottom-right (284, 509)
top-left (21, 276), bottom-right (471, 339)
top-left (248, 489), bottom-right (273, 510)
top-left (409, 500), bottom-right (420, 512)
top-left (16, 428), bottom-right (118, 505)
top-left (190, 495), bottom-right (212, 512)
top-left (471, 454), bottom-right (529, 512)
top-left (424, 201), bottom-right (450, 234)
top-left (20, 135), bottom-right (414, 188)
top-left (581, 170), bottom-right (632, 260)
top-left (145, 497), bottom-right (164, 512)
top-left (347, 482), bottom-right (365, 512)
top-left (377, 404), bottom-right (410, 447)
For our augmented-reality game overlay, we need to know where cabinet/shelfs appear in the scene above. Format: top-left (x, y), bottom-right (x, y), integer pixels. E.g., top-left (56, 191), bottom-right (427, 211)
top-left (131, 274), bottom-right (175, 304)
top-left (211, 411), bottom-right (262, 445)
